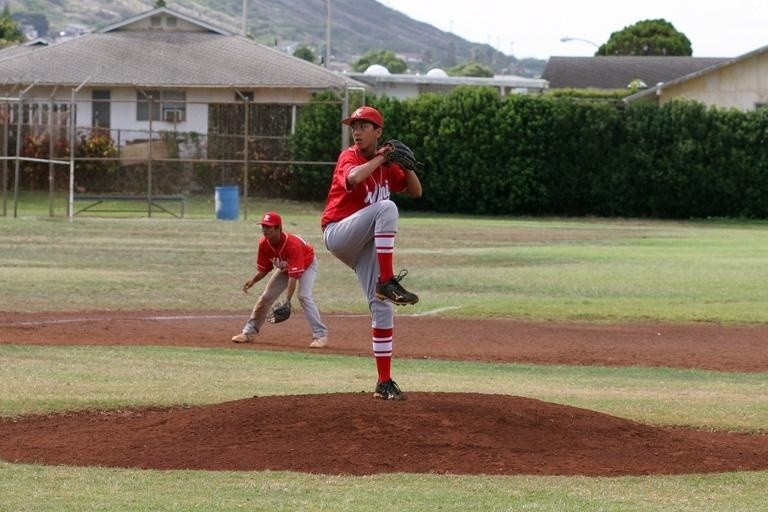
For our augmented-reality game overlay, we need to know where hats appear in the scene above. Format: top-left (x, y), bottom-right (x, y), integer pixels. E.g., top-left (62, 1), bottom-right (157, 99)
top-left (340, 105), bottom-right (385, 128)
top-left (256, 211), bottom-right (282, 227)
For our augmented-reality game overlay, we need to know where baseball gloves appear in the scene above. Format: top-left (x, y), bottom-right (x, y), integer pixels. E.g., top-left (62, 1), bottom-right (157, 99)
top-left (267, 303), bottom-right (291, 324)
top-left (376, 139), bottom-right (416, 170)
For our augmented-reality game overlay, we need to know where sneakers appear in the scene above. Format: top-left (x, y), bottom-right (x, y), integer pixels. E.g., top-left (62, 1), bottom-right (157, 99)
top-left (309, 335), bottom-right (328, 348)
top-left (374, 276), bottom-right (419, 305)
top-left (231, 333), bottom-right (257, 343)
top-left (372, 377), bottom-right (407, 401)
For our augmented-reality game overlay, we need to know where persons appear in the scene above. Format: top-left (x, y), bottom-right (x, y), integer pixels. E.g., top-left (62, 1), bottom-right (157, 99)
top-left (321, 106), bottom-right (424, 401)
top-left (231, 211), bottom-right (330, 349)
